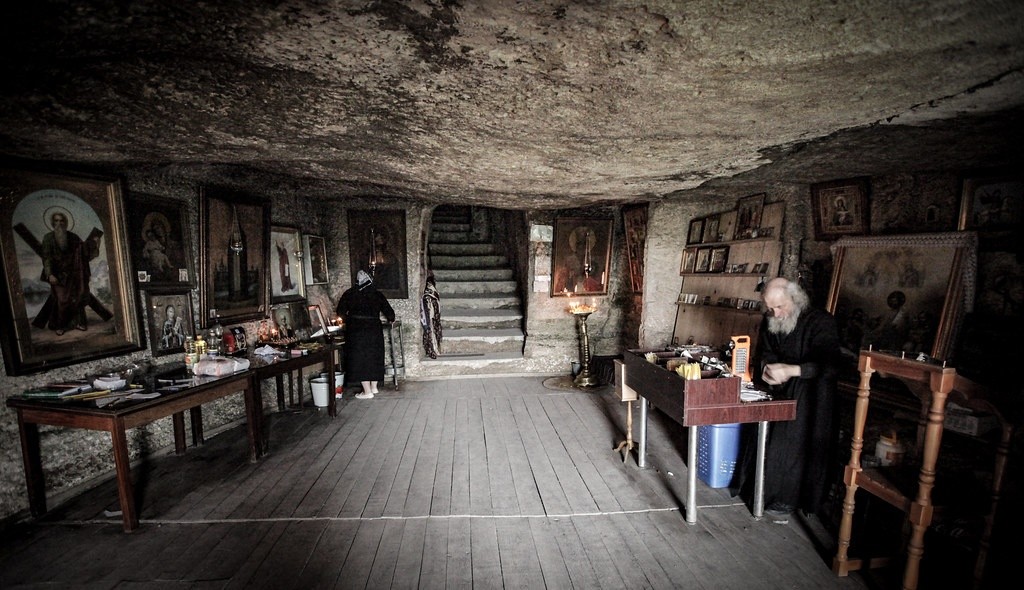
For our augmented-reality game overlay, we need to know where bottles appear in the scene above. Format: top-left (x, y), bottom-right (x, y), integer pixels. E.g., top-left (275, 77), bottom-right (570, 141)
top-left (183, 320), bottom-right (246, 375)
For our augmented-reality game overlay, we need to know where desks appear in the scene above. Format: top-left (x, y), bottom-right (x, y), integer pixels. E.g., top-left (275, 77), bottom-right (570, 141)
top-left (191, 343), bottom-right (342, 463)
top-left (624, 344), bottom-right (797, 525)
top-left (6, 366), bottom-right (258, 535)
top-left (382, 321), bottom-right (406, 391)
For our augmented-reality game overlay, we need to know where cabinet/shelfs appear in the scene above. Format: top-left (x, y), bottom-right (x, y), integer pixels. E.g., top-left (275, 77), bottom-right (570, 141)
top-left (674, 235), bottom-right (775, 316)
top-left (832, 351), bottom-right (1012, 590)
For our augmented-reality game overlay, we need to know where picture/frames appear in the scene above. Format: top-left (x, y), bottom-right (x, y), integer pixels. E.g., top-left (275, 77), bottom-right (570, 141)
top-left (958, 173), bottom-right (1024, 240)
top-left (146, 292), bottom-right (196, 357)
top-left (0, 165), bottom-right (142, 376)
top-left (269, 225), bottom-right (330, 340)
top-left (824, 237), bottom-right (965, 410)
top-left (680, 192), bottom-right (767, 274)
top-left (820, 380), bottom-right (996, 552)
top-left (121, 192), bottom-right (197, 291)
top-left (347, 208), bottom-right (408, 299)
top-left (550, 217), bottom-right (615, 298)
top-left (199, 185), bottom-right (272, 329)
top-left (810, 177), bottom-right (871, 239)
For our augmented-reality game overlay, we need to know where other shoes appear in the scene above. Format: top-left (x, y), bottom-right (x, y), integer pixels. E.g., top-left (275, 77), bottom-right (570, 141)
top-left (355, 392), bottom-right (374, 400)
top-left (763, 502), bottom-right (799, 520)
top-left (371, 388), bottom-right (379, 394)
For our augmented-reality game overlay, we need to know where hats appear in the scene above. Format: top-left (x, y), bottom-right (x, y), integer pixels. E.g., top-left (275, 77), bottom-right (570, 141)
top-left (355, 270), bottom-right (373, 290)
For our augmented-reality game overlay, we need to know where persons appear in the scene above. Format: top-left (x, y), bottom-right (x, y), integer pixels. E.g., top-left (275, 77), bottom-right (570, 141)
top-left (738, 277), bottom-right (842, 518)
top-left (336, 268), bottom-right (396, 399)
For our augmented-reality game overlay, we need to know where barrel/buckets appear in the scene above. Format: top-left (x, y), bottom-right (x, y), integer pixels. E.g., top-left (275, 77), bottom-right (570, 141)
top-left (321, 372), bottom-right (344, 398)
top-left (875, 427), bottom-right (906, 467)
top-left (308, 378), bottom-right (330, 407)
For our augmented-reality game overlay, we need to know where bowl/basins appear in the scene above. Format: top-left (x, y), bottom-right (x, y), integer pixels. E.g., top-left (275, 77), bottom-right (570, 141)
top-left (91, 360), bottom-right (147, 391)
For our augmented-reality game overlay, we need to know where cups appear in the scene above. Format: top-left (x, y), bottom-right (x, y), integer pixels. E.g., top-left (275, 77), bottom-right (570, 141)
top-left (679, 294), bottom-right (698, 304)
